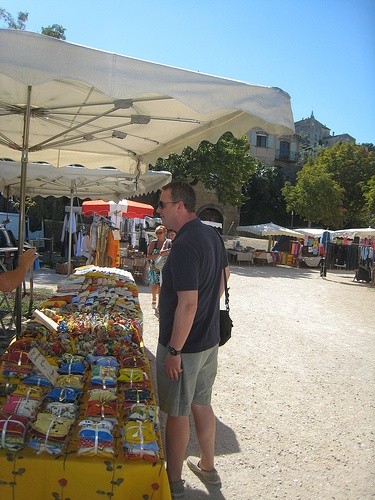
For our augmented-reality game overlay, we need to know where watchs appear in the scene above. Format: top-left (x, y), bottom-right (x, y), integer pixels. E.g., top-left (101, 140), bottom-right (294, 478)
top-left (168, 346), bottom-right (182, 355)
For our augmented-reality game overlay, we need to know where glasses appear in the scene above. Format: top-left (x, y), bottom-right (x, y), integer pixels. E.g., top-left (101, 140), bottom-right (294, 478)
top-left (156, 232), bottom-right (163, 235)
top-left (158, 200), bottom-right (186, 208)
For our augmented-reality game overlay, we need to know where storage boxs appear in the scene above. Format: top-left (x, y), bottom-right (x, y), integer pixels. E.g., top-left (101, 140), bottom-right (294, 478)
top-left (56, 262), bottom-right (72, 274)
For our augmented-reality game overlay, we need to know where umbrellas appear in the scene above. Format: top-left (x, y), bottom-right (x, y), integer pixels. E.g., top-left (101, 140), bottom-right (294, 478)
top-left (0, 160), bottom-right (172, 276)
top-left (236, 222), bottom-right (306, 252)
top-left (0, 28), bottom-right (296, 335)
top-left (82, 198), bottom-right (154, 223)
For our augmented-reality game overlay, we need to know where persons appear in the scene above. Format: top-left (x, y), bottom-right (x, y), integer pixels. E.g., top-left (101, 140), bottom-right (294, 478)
top-left (155, 181), bottom-right (230, 497)
top-left (0, 248), bottom-right (39, 292)
top-left (148, 225), bottom-right (167, 307)
top-left (160, 229), bottom-right (176, 286)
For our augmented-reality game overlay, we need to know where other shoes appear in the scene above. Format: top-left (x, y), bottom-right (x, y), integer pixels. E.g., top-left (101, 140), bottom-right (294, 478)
top-left (187, 456), bottom-right (221, 484)
top-left (170, 480), bottom-right (185, 497)
top-left (152, 298), bottom-right (156, 308)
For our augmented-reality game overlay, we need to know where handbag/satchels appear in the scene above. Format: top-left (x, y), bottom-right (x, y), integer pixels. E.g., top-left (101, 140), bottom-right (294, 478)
top-left (219, 309), bottom-right (233, 347)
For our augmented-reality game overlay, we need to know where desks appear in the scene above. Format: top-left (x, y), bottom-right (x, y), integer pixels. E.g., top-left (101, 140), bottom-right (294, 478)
top-left (295, 257), bottom-right (325, 268)
top-left (253, 252), bottom-right (267, 266)
top-left (228, 249), bottom-right (252, 266)
top-left (0, 265), bottom-right (172, 500)
top-left (122, 257), bottom-right (146, 282)
top-left (266, 252), bottom-right (282, 267)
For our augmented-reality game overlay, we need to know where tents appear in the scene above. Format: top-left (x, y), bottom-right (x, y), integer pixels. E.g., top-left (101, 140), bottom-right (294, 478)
top-left (294, 229), bottom-right (375, 276)
